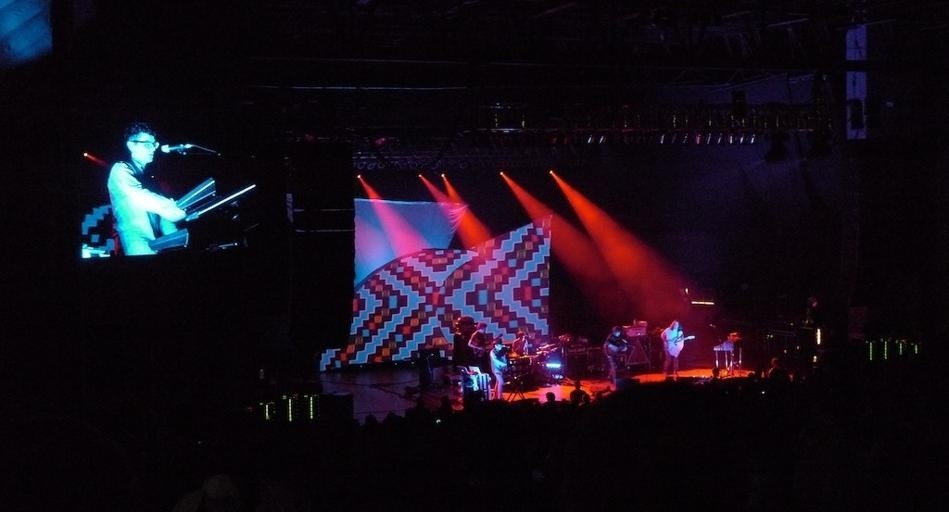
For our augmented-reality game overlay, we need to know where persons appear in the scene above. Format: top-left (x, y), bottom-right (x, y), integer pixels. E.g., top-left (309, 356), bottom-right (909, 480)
top-left (660, 320), bottom-right (684, 378)
top-left (603, 326), bottom-right (628, 382)
top-left (490, 341), bottom-right (508, 399)
top-left (512, 330), bottom-right (529, 356)
top-left (3, 359), bottom-right (949, 512)
top-left (468, 323), bottom-right (490, 359)
top-left (108, 121), bottom-right (185, 255)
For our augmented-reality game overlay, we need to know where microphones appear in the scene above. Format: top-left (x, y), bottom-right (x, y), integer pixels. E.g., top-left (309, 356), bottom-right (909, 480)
top-left (160, 142), bottom-right (193, 153)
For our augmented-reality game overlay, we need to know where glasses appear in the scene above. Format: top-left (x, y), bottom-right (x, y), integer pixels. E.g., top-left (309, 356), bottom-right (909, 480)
top-left (132, 140), bottom-right (160, 149)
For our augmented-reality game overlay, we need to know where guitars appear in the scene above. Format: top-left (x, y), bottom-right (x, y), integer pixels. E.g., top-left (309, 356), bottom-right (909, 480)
top-left (607, 343), bottom-right (632, 355)
top-left (482, 334), bottom-right (503, 351)
top-left (666, 335), bottom-right (695, 350)
